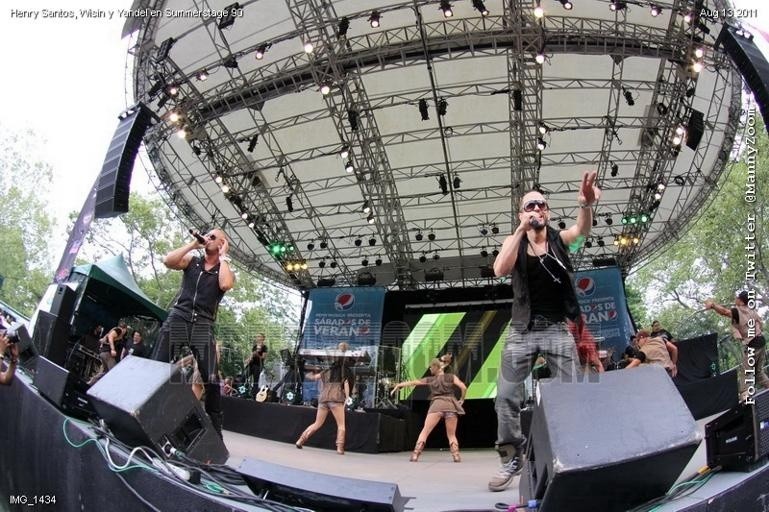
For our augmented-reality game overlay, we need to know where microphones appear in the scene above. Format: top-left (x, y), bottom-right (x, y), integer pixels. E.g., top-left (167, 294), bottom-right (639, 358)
top-left (527, 215), bottom-right (543, 229)
top-left (189, 228), bottom-right (206, 245)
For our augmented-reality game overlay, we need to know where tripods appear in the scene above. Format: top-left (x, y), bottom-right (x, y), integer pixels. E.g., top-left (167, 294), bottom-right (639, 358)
top-left (366, 370), bottom-right (398, 409)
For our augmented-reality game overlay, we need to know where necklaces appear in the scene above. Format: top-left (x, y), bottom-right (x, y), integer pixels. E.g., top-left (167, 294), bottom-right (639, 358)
top-left (529, 239), bottom-right (568, 272)
top-left (528, 242), bottom-right (563, 285)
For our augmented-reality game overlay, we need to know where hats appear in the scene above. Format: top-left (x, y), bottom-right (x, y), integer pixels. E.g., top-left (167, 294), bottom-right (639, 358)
top-left (736, 289), bottom-right (749, 304)
top-left (633, 331), bottom-right (649, 344)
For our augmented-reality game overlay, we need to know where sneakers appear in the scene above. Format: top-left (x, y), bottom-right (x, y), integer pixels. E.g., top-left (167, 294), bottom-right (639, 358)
top-left (488, 454), bottom-right (525, 492)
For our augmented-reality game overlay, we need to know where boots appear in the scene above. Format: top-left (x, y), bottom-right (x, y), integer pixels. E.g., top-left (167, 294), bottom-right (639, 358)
top-left (296, 425), bottom-right (315, 449)
top-left (450, 441), bottom-right (461, 463)
top-left (408, 441), bottom-right (426, 462)
top-left (336, 430), bottom-right (345, 455)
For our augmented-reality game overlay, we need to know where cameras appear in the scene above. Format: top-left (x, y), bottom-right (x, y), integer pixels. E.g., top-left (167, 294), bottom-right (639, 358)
top-left (1, 329), bottom-right (20, 347)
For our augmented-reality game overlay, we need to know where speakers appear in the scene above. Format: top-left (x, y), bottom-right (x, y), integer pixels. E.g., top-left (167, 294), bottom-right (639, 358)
top-left (49, 284), bottom-right (76, 321)
top-left (34, 353), bottom-right (92, 416)
top-left (706, 388), bottom-right (769, 473)
top-left (86, 355), bottom-right (230, 473)
top-left (720, 28), bottom-right (769, 134)
top-left (6, 321), bottom-right (40, 368)
top-left (525, 363), bottom-right (703, 511)
top-left (94, 108), bottom-right (152, 219)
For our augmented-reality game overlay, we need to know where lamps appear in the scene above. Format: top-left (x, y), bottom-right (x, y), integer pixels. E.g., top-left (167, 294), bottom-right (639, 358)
top-left (152, 40), bottom-right (269, 110)
top-left (302, 41), bottom-right (381, 225)
top-left (420, 96), bottom-right (464, 196)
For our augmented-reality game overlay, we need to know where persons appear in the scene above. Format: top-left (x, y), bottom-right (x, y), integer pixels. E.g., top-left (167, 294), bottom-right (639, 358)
top-left (487, 171), bottom-right (602, 491)
top-left (389, 354), bottom-right (468, 463)
top-left (149, 229), bottom-right (238, 441)
top-left (1, 333), bottom-right (21, 387)
top-left (532, 308), bottom-right (679, 385)
top-left (704, 291), bottom-right (768, 404)
top-left (83, 319), bottom-right (356, 456)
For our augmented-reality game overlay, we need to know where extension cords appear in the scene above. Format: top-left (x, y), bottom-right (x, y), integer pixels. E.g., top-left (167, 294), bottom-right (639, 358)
top-left (152, 457), bottom-right (201, 485)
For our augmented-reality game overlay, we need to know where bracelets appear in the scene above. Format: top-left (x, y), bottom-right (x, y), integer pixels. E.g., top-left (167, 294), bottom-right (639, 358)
top-left (711, 303), bottom-right (715, 308)
top-left (220, 256), bottom-right (232, 264)
top-left (1, 352), bottom-right (4, 360)
top-left (10, 358), bottom-right (19, 366)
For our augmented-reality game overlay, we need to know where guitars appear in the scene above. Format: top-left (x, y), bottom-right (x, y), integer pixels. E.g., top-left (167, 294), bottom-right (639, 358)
top-left (254, 384), bottom-right (270, 403)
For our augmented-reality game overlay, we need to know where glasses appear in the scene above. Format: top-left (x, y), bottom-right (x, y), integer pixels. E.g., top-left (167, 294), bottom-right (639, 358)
top-left (523, 200), bottom-right (548, 212)
top-left (204, 234), bottom-right (220, 240)
